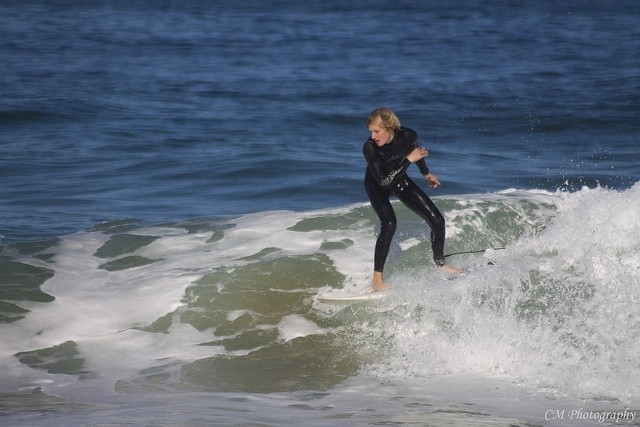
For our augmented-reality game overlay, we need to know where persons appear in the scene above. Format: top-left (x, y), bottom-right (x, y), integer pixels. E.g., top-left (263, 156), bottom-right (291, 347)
top-left (362, 107), bottom-right (467, 291)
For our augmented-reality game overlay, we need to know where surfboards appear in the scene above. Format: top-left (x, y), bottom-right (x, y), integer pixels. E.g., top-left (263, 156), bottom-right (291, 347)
top-left (318, 262), bottom-right (498, 301)
